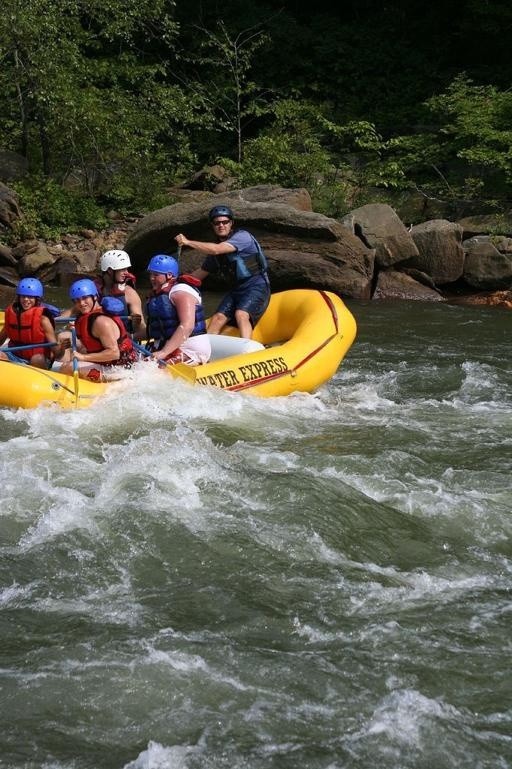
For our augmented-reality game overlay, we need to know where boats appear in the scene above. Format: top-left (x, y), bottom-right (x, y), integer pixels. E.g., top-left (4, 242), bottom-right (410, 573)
top-left (0, 287), bottom-right (358, 414)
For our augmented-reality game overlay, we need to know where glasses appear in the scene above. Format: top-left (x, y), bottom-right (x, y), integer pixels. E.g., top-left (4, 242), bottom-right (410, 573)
top-left (211, 220), bottom-right (231, 226)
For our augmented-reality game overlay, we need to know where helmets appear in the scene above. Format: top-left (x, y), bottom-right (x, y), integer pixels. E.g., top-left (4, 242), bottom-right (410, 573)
top-left (146, 254), bottom-right (179, 281)
top-left (208, 205), bottom-right (233, 222)
top-left (16, 277), bottom-right (44, 297)
top-left (100, 249), bottom-right (133, 272)
top-left (69, 279), bottom-right (99, 300)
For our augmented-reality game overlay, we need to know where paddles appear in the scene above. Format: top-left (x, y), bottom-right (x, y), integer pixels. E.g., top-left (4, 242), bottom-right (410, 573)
top-left (130, 339), bottom-right (195, 388)
top-left (73, 328), bottom-right (81, 410)
top-left (1, 312), bottom-right (133, 333)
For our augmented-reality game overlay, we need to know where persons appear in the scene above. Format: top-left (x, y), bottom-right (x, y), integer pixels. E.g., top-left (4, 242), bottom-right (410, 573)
top-left (174, 206), bottom-right (271, 338)
top-left (57, 250), bottom-right (146, 346)
top-left (0, 278), bottom-right (70, 369)
top-left (131, 254), bottom-right (211, 369)
top-left (61, 278), bottom-right (137, 381)
